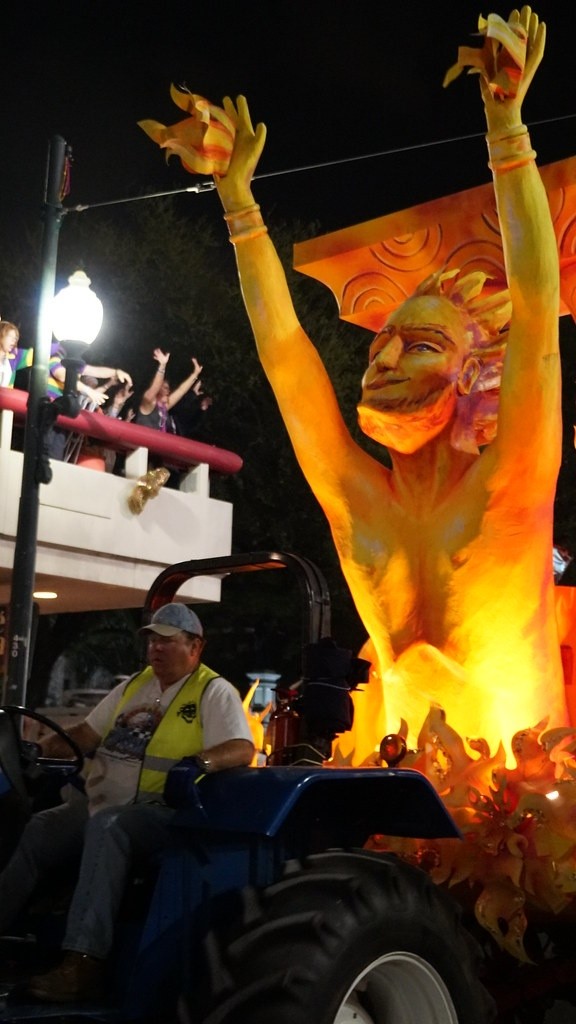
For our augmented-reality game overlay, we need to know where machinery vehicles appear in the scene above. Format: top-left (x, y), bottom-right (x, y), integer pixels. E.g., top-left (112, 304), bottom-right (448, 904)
top-left (0, 550), bottom-right (514, 1023)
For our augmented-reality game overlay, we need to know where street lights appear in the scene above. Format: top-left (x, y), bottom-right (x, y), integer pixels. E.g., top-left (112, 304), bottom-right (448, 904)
top-left (0, 267), bottom-right (103, 731)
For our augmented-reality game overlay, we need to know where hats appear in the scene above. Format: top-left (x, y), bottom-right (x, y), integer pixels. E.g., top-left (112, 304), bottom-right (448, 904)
top-left (136, 604), bottom-right (203, 638)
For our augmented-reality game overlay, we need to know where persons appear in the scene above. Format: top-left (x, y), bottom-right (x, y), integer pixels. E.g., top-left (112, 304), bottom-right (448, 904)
top-left (0, 316), bottom-right (205, 488)
top-left (192, 7), bottom-right (570, 818)
top-left (2, 603), bottom-right (255, 1001)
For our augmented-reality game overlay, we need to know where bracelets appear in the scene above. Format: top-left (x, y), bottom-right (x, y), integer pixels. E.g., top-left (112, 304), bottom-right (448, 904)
top-left (158, 368), bottom-right (165, 373)
top-left (201, 757), bottom-right (211, 774)
top-left (222, 203), bottom-right (268, 243)
top-left (484, 124), bottom-right (537, 170)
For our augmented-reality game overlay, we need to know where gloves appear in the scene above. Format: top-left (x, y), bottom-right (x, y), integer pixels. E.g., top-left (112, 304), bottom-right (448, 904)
top-left (162, 756), bottom-right (205, 805)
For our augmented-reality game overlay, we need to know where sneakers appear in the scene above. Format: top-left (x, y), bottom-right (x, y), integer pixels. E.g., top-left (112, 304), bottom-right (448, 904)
top-left (25, 955), bottom-right (106, 1003)
